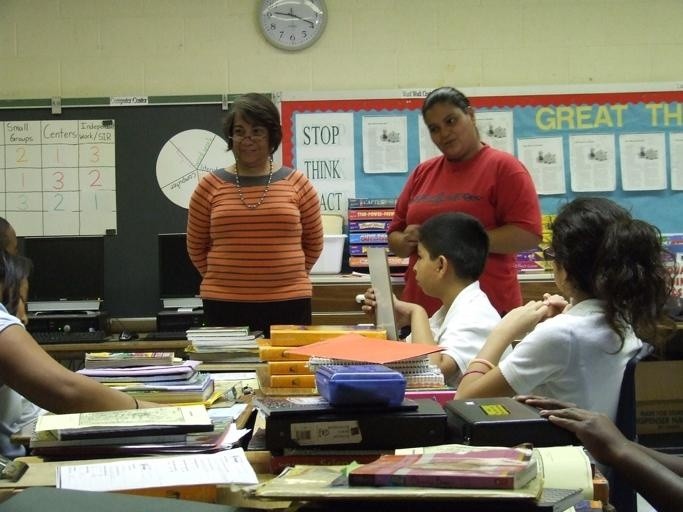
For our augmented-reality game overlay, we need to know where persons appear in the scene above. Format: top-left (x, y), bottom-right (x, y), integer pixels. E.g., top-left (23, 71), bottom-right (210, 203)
top-left (511, 394), bottom-right (683, 511)
top-left (361, 212), bottom-right (514, 390)
top-left (385, 86), bottom-right (543, 338)
top-left (186, 92), bottom-right (325, 340)
top-left (453, 196), bottom-right (679, 445)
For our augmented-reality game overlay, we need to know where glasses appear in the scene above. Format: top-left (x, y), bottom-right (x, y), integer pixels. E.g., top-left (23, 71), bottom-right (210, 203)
top-left (227, 132), bottom-right (271, 142)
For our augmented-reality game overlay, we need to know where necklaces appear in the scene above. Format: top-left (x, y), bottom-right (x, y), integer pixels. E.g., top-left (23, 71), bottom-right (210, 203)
top-left (234, 157), bottom-right (273, 209)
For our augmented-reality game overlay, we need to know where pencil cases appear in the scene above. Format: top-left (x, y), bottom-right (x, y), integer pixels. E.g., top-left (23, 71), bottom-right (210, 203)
top-left (317, 364), bottom-right (406, 407)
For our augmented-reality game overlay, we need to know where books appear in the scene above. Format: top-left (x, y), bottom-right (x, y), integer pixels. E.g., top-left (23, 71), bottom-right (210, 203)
top-left (653, 233), bottom-right (683, 321)
top-left (348, 198), bottom-right (559, 281)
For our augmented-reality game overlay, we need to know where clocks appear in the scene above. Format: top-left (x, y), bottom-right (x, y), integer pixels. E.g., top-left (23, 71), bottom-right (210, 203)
top-left (259, 1), bottom-right (327, 52)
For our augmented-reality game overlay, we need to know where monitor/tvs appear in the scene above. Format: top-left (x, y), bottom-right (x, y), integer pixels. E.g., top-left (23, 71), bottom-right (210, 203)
top-left (158, 233), bottom-right (204, 311)
top-left (18, 236), bottom-right (106, 316)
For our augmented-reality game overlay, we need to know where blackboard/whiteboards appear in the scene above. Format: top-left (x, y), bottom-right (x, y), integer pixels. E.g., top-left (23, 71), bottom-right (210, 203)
top-left (0, 118), bottom-right (119, 239)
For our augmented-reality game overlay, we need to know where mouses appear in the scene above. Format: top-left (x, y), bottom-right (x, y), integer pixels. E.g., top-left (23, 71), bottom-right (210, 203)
top-left (118, 328), bottom-right (134, 341)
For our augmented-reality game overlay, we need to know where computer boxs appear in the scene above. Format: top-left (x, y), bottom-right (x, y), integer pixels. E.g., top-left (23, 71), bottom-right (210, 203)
top-left (28, 313), bottom-right (109, 333)
top-left (155, 309), bottom-right (203, 331)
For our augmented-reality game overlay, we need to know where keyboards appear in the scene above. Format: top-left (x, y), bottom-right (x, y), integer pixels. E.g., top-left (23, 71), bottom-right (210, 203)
top-left (28, 330), bottom-right (104, 343)
top-left (145, 332), bottom-right (187, 340)
